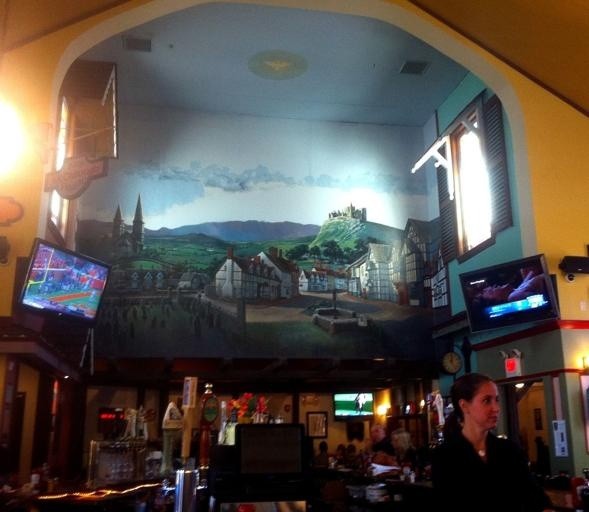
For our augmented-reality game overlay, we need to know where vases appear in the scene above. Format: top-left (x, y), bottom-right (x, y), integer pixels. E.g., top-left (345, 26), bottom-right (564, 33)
top-left (237, 413), bottom-right (268, 424)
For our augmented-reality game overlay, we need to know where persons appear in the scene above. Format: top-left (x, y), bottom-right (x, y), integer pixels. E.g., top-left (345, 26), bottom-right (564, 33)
top-left (469, 266), bottom-right (546, 310)
top-left (28, 243), bottom-right (108, 312)
top-left (534, 436), bottom-right (550, 476)
top-left (312, 423), bottom-right (424, 481)
top-left (429, 372), bottom-right (556, 512)
top-left (145, 432), bottom-right (201, 479)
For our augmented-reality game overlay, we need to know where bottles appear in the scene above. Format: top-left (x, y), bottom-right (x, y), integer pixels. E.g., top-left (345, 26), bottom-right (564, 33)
top-left (30, 462), bottom-right (53, 495)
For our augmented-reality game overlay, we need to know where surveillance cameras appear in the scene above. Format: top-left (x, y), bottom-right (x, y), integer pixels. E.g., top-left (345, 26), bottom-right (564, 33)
top-left (565, 272), bottom-right (575, 283)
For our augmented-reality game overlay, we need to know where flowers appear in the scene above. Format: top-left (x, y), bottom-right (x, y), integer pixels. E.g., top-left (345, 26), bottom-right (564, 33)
top-left (228, 391), bottom-right (271, 420)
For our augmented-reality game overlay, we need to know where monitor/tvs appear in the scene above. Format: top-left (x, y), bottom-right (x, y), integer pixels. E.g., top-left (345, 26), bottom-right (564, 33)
top-left (459, 254), bottom-right (560, 335)
top-left (16, 237), bottom-right (113, 328)
top-left (332, 392), bottom-right (375, 421)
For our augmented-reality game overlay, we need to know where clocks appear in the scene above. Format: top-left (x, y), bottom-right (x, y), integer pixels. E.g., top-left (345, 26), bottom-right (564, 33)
top-left (441, 351), bottom-right (462, 375)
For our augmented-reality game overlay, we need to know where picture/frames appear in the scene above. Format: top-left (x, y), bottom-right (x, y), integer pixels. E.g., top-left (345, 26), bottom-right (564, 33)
top-left (306, 411), bottom-right (328, 439)
